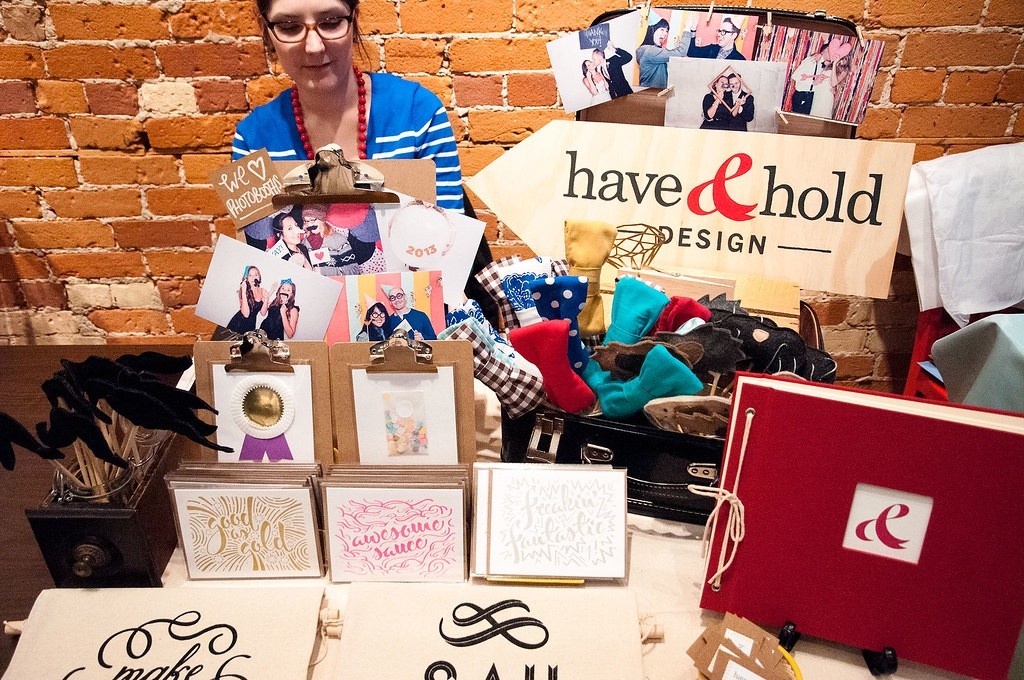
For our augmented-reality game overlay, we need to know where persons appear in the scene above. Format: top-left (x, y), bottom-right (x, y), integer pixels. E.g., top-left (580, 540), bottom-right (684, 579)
top-left (231, 1), bottom-right (466, 302)
top-left (583, 13), bottom-right (850, 132)
top-left (228, 204), bottom-right (435, 341)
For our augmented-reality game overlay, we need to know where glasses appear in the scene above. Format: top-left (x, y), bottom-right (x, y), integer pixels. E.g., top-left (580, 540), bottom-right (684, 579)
top-left (302, 218), bottom-right (318, 224)
top-left (261, 6), bottom-right (355, 44)
top-left (716, 29), bottom-right (736, 35)
top-left (389, 292), bottom-right (405, 301)
top-left (370, 312), bottom-right (386, 318)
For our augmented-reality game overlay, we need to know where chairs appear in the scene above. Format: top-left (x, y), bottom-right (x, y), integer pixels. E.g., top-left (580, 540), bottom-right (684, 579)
top-left (903, 143), bottom-right (1024, 402)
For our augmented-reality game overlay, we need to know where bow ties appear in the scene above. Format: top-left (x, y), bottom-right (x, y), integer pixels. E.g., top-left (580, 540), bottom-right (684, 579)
top-left (437, 219), bottom-right (713, 420)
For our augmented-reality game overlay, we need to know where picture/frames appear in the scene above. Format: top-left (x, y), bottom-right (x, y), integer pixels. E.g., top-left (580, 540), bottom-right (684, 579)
top-left (1, 143), bottom-right (628, 680)
